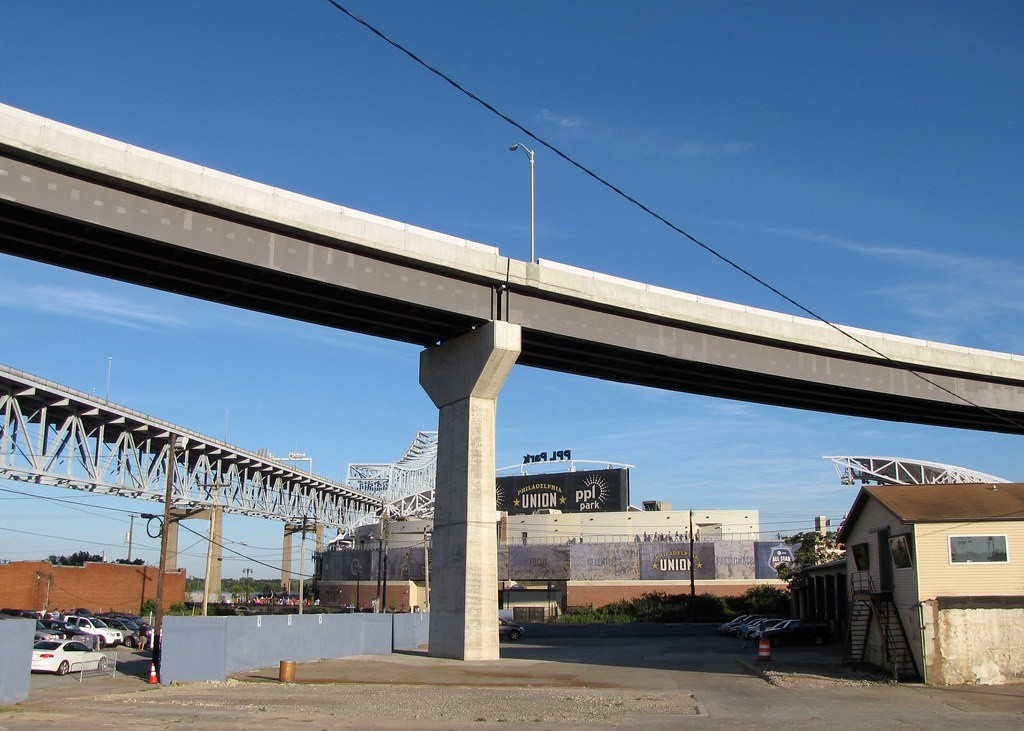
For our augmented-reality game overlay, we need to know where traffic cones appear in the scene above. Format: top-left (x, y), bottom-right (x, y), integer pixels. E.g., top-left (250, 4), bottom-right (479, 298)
top-left (147, 662), bottom-right (160, 684)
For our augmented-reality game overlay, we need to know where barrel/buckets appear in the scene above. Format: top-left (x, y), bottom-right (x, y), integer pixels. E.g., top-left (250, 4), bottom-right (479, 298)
top-left (279, 660), bottom-right (296, 683)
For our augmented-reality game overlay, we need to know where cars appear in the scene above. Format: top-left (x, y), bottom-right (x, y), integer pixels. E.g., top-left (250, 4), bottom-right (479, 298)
top-left (718, 614), bottom-right (801, 645)
top-left (498, 617), bottom-right (525, 641)
top-left (30, 640), bottom-right (109, 676)
top-left (0, 607), bottom-right (156, 650)
top-left (225, 605), bottom-right (256, 617)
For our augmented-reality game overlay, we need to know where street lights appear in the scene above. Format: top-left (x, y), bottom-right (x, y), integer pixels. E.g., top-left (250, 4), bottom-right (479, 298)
top-left (509, 142), bottom-right (536, 263)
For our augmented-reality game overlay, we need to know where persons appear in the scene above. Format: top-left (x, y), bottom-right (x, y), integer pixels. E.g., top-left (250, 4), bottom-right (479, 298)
top-left (44, 608), bottom-right (65, 621)
top-left (138, 622), bottom-right (149, 651)
top-left (737, 618), bottom-right (765, 640)
